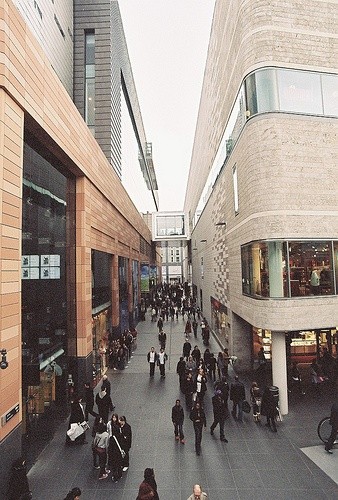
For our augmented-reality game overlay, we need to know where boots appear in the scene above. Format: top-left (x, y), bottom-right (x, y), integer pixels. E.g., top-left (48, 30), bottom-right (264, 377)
top-left (257, 413), bottom-right (260, 420)
top-left (253, 414), bottom-right (257, 422)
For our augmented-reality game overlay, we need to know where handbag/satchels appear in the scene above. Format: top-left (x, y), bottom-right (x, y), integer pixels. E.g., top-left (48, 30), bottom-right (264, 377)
top-left (121, 450), bottom-right (126, 458)
top-left (96, 447), bottom-right (106, 454)
top-left (255, 400), bottom-right (261, 406)
top-left (242, 400), bottom-right (251, 413)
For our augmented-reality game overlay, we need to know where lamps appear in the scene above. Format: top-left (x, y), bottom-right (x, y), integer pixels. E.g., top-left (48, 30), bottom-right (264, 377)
top-left (193, 248), bottom-right (197, 250)
top-left (201, 240), bottom-right (206, 242)
top-left (217, 222), bottom-right (226, 225)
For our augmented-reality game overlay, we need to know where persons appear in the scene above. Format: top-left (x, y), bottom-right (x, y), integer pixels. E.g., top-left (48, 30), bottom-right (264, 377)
top-left (172, 399), bottom-right (184, 444)
top-left (292, 268), bottom-right (328, 295)
top-left (90, 414), bottom-right (132, 484)
top-left (187, 484), bottom-right (208, 500)
top-left (288, 362), bottom-right (305, 395)
top-left (210, 389), bottom-right (229, 442)
top-left (285, 335), bottom-right (292, 359)
top-left (8, 457), bottom-right (32, 500)
top-left (189, 401), bottom-right (206, 456)
top-left (230, 375), bottom-right (280, 432)
top-left (136, 483), bottom-right (155, 500)
top-left (325, 399), bottom-right (338, 453)
top-left (65, 374), bottom-right (115, 445)
top-left (176, 339), bottom-right (231, 409)
top-left (139, 282), bottom-right (210, 378)
top-left (141, 468), bottom-right (159, 500)
top-left (309, 331), bottom-right (338, 392)
top-left (107, 328), bottom-right (138, 371)
top-left (256, 347), bottom-right (266, 371)
top-left (64, 487), bottom-right (81, 500)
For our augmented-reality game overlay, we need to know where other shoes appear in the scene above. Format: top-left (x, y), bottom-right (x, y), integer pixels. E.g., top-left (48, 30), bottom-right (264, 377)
top-left (106, 470), bottom-right (111, 474)
top-left (180, 440), bottom-right (184, 444)
top-left (99, 474), bottom-right (108, 479)
top-left (210, 430), bottom-right (214, 435)
top-left (94, 466), bottom-right (102, 469)
top-left (122, 467), bottom-right (128, 471)
top-left (328, 450), bottom-right (332, 453)
top-left (223, 439), bottom-right (228, 443)
top-left (176, 436), bottom-right (179, 440)
